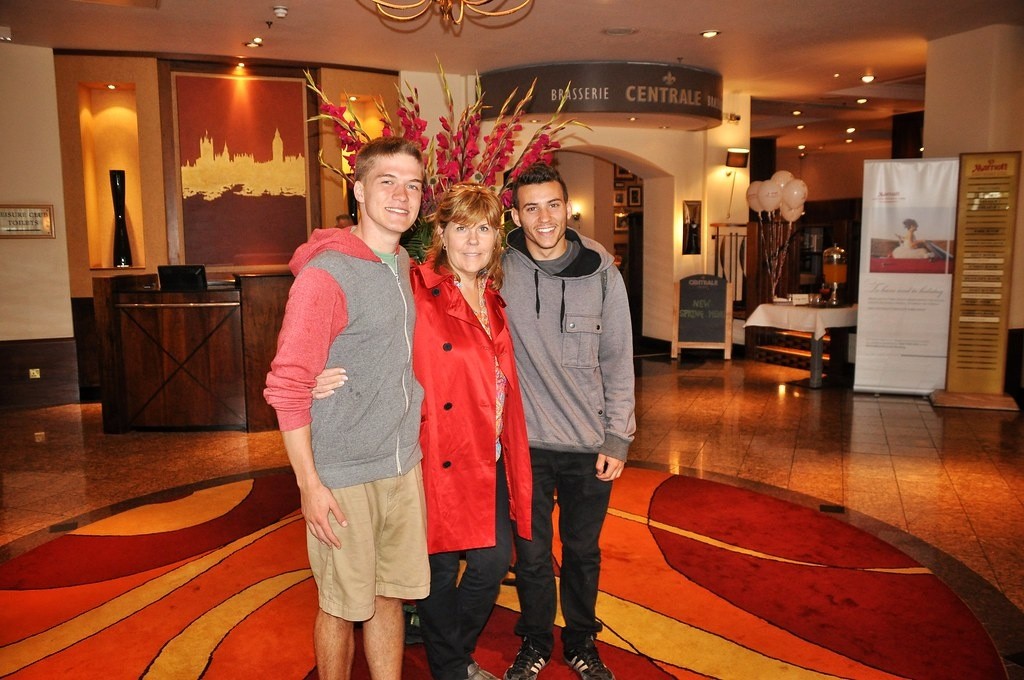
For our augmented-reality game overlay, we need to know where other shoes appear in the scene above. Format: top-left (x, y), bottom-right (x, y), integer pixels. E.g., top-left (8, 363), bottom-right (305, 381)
top-left (467, 660), bottom-right (500, 680)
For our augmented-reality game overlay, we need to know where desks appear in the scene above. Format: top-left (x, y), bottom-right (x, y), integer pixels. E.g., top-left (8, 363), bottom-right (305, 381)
top-left (742, 302), bottom-right (861, 388)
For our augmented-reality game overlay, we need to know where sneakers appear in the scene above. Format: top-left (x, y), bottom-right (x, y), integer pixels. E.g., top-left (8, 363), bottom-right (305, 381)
top-left (563, 639), bottom-right (615, 680)
top-left (505, 637), bottom-right (554, 680)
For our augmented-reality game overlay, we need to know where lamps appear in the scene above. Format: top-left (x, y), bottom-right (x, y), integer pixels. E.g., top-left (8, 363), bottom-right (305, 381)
top-left (726, 148), bottom-right (750, 168)
top-left (573, 208), bottom-right (580, 219)
top-left (372, 0), bottom-right (530, 26)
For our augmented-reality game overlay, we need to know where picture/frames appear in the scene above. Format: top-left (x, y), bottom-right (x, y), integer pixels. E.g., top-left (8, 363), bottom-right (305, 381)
top-left (682, 201), bottom-right (703, 256)
top-left (614, 165), bottom-right (637, 182)
top-left (615, 214), bottom-right (631, 232)
top-left (614, 190), bottom-right (627, 207)
top-left (627, 186), bottom-right (641, 206)
top-left (156, 59), bottom-right (322, 279)
top-left (614, 181), bottom-right (625, 191)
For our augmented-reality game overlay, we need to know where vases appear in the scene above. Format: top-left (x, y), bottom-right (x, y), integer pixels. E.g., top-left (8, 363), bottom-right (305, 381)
top-left (110, 170), bottom-right (133, 267)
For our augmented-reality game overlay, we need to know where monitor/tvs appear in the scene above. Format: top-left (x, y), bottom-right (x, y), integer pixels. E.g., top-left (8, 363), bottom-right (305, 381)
top-left (158, 264), bottom-right (207, 291)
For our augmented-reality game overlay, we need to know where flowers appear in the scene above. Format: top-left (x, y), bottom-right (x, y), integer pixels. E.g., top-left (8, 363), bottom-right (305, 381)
top-left (298, 54), bottom-right (597, 257)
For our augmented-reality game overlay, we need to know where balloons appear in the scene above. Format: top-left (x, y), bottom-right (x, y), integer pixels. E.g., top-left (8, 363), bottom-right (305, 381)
top-left (747, 170), bottom-right (808, 222)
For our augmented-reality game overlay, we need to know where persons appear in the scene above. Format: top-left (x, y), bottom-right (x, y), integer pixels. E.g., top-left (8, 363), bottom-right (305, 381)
top-left (312, 183), bottom-right (533, 680)
top-left (263, 136), bottom-right (431, 680)
top-left (497, 160), bottom-right (636, 680)
top-left (892, 219), bottom-right (927, 258)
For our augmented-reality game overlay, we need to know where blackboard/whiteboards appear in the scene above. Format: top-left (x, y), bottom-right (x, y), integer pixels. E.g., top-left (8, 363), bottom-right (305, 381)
top-left (672, 274), bottom-right (733, 349)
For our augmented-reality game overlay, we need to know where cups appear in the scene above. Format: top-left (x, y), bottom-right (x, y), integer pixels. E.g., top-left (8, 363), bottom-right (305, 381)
top-left (809, 294), bottom-right (822, 304)
